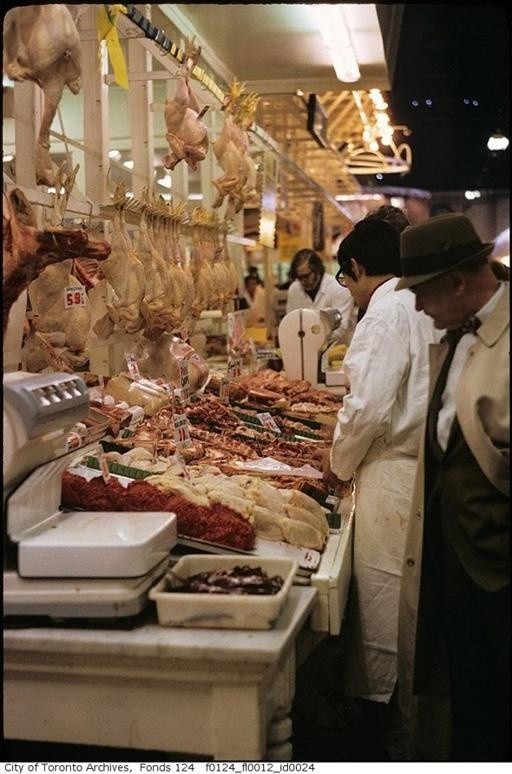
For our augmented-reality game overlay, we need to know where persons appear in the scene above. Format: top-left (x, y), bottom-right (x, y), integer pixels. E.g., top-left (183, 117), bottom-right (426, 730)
top-left (395, 213), bottom-right (511, 761)
top-left (367, 204), bottom-right (411, 230)
top-left (286, 248), bottom-right (357, 383)
top-left (489, 259), bottom-right (510, 281)
top-left (248, 267), bottom-right (264, 287)
top-left (324, 217), bottom-right (446, 722)
top-left (282, 272), bottom-right (294, 290)
top-left (242, 276), bottom-right (265, 309)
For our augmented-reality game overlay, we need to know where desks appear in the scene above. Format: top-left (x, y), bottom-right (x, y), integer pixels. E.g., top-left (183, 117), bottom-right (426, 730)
top-left (68, 434), bottom-right (355, 644)
top-left (3, 588), bottom-right (320, 762)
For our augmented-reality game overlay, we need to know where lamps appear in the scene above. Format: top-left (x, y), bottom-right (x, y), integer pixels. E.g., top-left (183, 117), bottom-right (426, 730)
top-left (313, 2), bottom-right (361, 83)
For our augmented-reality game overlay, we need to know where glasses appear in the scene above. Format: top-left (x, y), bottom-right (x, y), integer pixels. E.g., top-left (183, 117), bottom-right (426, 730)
top-left (335, 256), bottom-right (361, 287)
top-left (297, 270), bottom-right (313, 279)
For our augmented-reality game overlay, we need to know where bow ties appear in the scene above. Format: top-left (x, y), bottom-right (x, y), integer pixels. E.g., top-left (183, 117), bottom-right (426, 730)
top-left (444, 317), bottom-right (481, 344)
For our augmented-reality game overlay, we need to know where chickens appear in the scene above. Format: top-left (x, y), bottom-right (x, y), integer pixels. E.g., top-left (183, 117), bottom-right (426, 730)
top-left (0, 2), bottom-right (86, 153)
top-left (1, 160), bottom-right (242, 344)
top-left (162, 39), bottom-right (208, 170)
top-left (211, 76), bottom-right (258, 212)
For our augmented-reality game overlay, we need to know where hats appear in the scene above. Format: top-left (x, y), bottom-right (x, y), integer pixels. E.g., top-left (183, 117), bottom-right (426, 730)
top-left (394, 212), bottom-right (494, 290)
